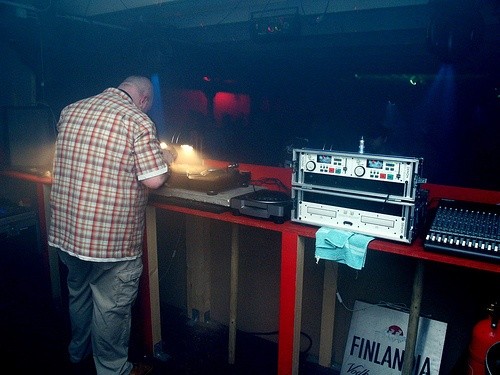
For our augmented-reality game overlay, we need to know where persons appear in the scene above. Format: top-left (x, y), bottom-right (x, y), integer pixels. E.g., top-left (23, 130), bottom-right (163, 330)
top-left (47, 74), bottom-right (173, 375)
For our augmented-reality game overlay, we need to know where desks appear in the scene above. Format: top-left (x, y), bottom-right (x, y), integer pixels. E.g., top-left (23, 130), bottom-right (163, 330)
top-left (0, 156), bottom-right (500, 375)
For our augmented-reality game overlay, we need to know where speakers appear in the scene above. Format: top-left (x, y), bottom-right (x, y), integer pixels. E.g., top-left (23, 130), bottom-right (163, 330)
top-left (0, 105), bottom-right (56, 171)
